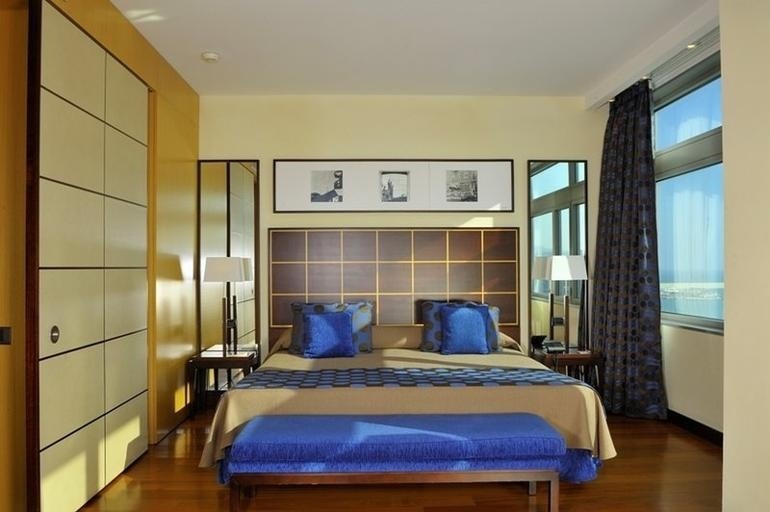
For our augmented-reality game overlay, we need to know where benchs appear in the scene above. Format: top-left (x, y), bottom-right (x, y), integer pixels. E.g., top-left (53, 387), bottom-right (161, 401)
top-left (226, 414), bottom-right (570, 512)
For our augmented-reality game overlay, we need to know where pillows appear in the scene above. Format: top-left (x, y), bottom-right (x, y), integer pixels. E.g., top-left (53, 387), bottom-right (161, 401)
top-left (289, 300), bottom-right (375, 355)
top-left (302, 310), bottom-right (355, 358)
top-left (416, 298), bottom-right (503, 355)
top-left (438, 304), bottom-right (492, 355)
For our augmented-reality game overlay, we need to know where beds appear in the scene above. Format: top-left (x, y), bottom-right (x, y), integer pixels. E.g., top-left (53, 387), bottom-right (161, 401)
top-left (197, 326), bottom-right (618, 490)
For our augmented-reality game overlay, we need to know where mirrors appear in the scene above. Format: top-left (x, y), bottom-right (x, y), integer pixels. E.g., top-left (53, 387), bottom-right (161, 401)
top-left (527, 159), bottom-right (589, 386)
top-left (197, 159), bottom-right (260, 396)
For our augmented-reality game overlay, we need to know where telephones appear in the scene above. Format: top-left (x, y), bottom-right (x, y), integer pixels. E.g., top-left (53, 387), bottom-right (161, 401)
top-left (542, 341), bottom-right (566, 353)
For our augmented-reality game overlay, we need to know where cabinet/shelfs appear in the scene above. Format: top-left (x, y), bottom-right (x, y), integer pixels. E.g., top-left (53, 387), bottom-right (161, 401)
top-left (229, 161), bottom-right (254, 345)
top-left (24, 0), bottom-right (149, 512)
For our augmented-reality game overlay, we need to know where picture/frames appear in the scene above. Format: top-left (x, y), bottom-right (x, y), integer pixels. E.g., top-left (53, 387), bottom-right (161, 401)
top-left (272, 158), bottom-right (515, 213)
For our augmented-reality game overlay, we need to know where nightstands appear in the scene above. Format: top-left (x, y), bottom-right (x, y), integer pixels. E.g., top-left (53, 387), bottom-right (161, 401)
top-left (205, 344), bottom-right (259, 374)
top-left (530, 342), bottom-right (578, 356)
top-left (185, 351), bottom-right (255, 420)
top-left (536, 348), bottom-right (604, 408)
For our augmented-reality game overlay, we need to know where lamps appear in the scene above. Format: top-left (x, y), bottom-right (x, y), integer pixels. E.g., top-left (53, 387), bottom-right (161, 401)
top-left (532, 256), bottom-right (559, 340)
top-left (203, 257), bottom-right (245, 358)
top-left (551, 255), bottom-right (588, 354)
top-left (230, 258), bottom-right (253, 355)
